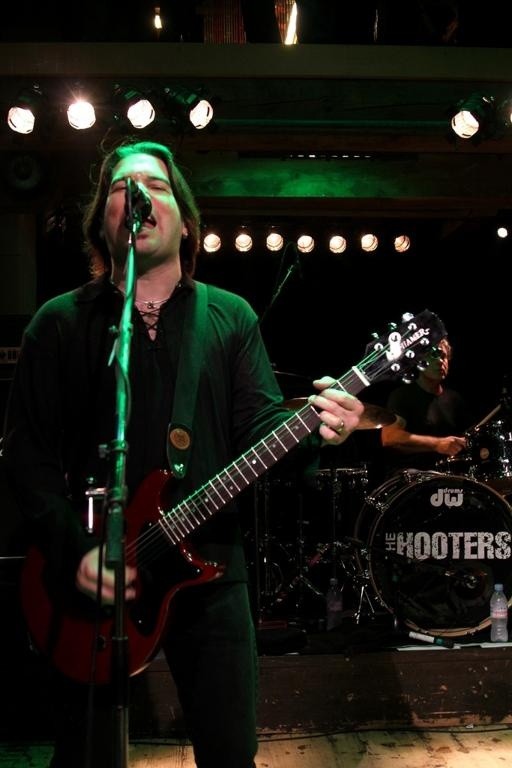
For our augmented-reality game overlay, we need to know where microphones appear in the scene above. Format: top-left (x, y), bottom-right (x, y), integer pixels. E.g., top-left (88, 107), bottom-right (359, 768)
top-left (125, 178), bottom-right (153, 221)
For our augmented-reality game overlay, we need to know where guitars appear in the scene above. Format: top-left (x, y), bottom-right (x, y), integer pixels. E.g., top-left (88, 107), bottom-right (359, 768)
top-left (23, 310), bottom-right (446, 685)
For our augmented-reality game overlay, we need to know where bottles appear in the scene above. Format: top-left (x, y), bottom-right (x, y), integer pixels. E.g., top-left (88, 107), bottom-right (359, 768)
top-left (489, 582), bottom-right (510, 645)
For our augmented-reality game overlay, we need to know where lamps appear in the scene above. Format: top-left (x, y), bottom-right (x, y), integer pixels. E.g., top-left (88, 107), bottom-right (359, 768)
top-left (5, 76), bottom-right (217, 139)
top-left (439, 86), bottom-right (511, 143)
top-left (200, 221), bottom-right (416, 259)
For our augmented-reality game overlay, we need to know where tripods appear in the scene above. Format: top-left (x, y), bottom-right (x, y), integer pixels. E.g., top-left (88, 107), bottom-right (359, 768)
top-left (281, 499), bottom-right (377, 624)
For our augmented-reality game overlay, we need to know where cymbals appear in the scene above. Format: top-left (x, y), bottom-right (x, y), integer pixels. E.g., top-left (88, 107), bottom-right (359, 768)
top-left (272, 397), bottom-right (397, 430)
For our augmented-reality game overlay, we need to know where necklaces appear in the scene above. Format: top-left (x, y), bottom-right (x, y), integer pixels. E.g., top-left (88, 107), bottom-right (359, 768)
top-left (118, 287), bottom-right (169, 309)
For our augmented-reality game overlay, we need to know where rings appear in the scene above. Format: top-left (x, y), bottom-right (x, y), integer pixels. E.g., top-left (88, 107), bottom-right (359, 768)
top-left (336, 422), bottom-right (345, 433)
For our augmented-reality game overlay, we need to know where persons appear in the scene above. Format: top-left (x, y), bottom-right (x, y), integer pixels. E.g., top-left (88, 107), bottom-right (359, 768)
top-left (380, 337), bottom-right (467, 529)
top-left (0, 141), bottom-right (366, 768)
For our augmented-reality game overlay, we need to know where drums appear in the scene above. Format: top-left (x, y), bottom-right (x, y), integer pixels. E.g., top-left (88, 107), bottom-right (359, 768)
top-left (466, 421), bottom-right (512, 482)
top-left (314, 468), bottom-right (368, 511)
top-left (437, 456), bottom-right (470, 476)
top-left (355, 471), bottom-right (511, 637)
